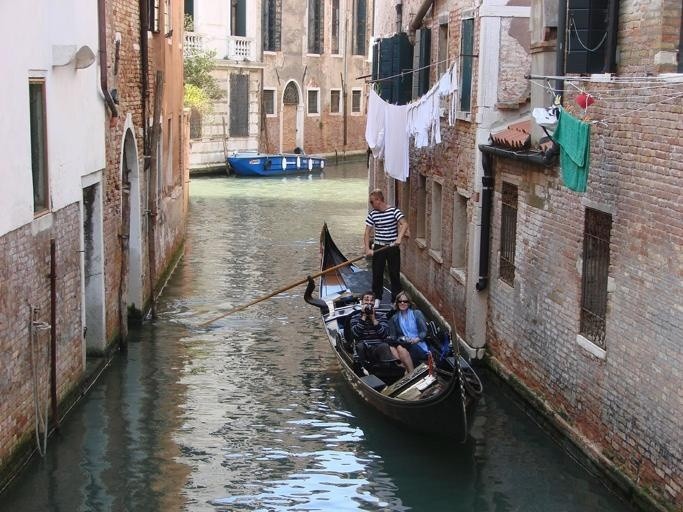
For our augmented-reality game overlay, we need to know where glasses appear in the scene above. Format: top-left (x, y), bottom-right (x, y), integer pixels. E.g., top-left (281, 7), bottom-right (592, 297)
top-left (370, 200), bottom-right (375, 203)
top-left (397, 300), bottom-right (409, 303)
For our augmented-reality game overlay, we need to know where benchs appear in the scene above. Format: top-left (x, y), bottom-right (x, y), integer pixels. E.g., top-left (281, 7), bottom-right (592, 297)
top-left (343, 310), bottom-right (396, 353)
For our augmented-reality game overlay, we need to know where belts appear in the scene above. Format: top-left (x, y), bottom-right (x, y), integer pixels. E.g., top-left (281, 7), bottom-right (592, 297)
top-left (374, 241), bottom-right (396, 247)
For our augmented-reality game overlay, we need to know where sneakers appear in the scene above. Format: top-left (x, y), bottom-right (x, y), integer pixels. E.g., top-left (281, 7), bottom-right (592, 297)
top-left (374, 299), bottom-right (381, 308)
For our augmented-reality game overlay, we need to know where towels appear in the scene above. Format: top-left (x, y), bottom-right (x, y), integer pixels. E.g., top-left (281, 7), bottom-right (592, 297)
top-left (549, 107), bottom-right (590, 193)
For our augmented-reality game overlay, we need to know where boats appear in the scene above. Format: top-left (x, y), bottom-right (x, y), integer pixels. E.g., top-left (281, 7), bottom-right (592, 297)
top-left (228, 153), bottom-right (327, 174)
top-left (305, 222), bottom-right (483, 445)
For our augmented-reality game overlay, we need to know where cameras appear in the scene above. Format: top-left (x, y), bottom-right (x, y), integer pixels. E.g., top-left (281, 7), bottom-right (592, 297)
top-left (364, 303), bottom-right (375, 316)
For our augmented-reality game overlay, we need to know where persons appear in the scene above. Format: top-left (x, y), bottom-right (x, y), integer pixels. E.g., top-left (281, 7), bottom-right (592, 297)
top-left (350, 292), bottom-right (407, 377)
top-left (362, 188), bottom-right (409, 306)
top-left (388, 292), bottom-right (430, 376)
